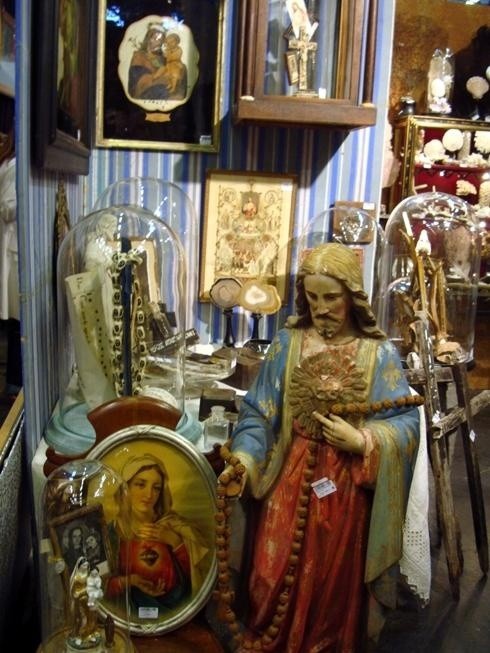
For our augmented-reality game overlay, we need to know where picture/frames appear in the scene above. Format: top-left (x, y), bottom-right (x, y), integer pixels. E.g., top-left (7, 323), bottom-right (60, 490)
top-left (32, 1), bottom-right (226, 175)
top-left (199, 168), bottom-right (297, 309)
top-left (47, 425), bottom-right (227, 637)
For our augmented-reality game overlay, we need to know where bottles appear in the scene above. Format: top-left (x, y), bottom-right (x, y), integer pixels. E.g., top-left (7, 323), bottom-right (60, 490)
top-left (200, 404), bottom-right (230, 451)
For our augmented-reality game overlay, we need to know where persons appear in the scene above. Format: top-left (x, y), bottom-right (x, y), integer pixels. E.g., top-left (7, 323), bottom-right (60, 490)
top-left (68, 555), bottom-right (98, 646)
top-left (102, 451), bottom-right (209, 622)
top-left (128, 19), bottom-right (187, 100)
top-left (80, 530), bottom-right (104, 568)
top-left (227, 242), bottom-right (422, 652)
top-left (152, 31), bottom-right (187, 95)
top-left (62, 520), bottom-right (90, 572)
top-left (86, 564), bottom-right (103, 610)
